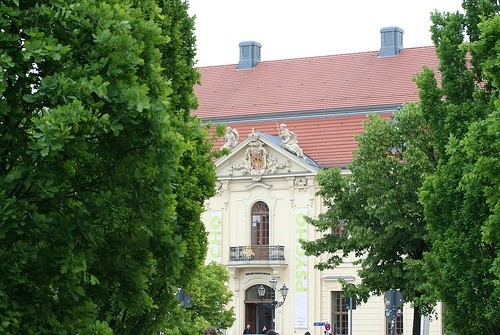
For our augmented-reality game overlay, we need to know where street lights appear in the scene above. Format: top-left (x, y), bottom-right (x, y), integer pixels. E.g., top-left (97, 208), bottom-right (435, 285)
top-left (258, 276), bottom-right (289, 335)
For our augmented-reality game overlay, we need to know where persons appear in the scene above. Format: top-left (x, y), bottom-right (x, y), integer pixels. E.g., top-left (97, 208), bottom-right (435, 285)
top-left (262, 325), bottom-right (268, 334)
top-left (267, 154), bottom-right (278, 173)
top-left (243, 324), bottom-right (253, 334)
top-left (266, 330), bottom-right (277, 335)
top-left (279, 124), bottom-right (304, 158)
top-left (205, 326), bottom-right (217, 335)
top-left (220, 127), bottom-right (236, 148)
